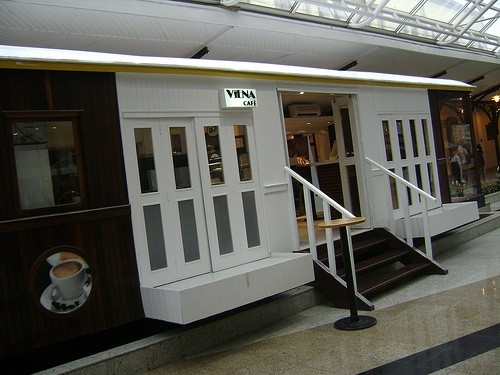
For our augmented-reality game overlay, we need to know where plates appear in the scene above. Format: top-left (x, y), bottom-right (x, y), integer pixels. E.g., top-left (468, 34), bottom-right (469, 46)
top-left (40, 272), bottom-right (93, 314)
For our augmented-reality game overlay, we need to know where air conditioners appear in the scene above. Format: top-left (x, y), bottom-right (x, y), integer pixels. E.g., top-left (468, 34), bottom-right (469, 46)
top-left (289, 104), bottom-right (322, 118)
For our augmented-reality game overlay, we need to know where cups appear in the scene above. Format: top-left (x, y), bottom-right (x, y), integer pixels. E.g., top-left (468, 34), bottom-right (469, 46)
top-left (49, 257), bottom-right (86, 302)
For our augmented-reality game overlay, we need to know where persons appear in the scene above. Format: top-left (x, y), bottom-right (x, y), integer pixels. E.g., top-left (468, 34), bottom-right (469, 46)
top-left (451, 151), bottom-right (466, 188)
top-left (476, 144), bottom-right (488, 182)
top-left (457, 145), bottom-right (469, 182)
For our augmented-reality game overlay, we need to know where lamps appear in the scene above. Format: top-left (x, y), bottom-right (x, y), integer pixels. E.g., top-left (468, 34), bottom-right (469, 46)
top-left (492, 95), bottom-right (500, 103)
